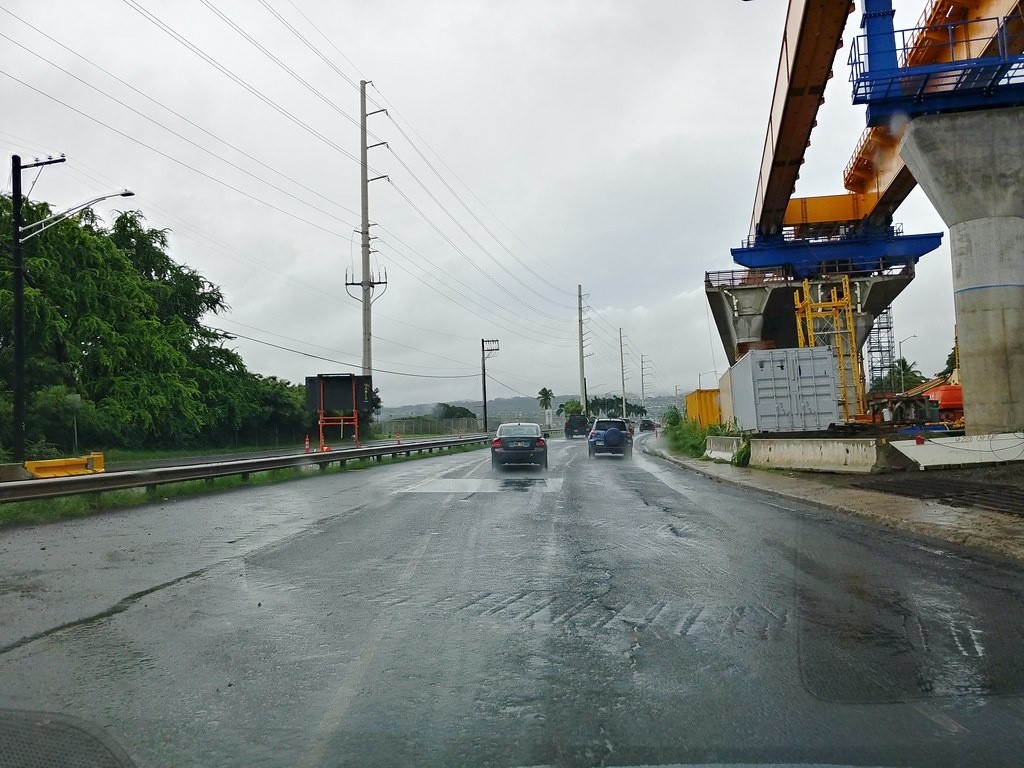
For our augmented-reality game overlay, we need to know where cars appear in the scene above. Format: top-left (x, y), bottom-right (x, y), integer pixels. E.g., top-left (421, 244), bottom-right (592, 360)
top-left (490, 423), bottom-right (550, 470)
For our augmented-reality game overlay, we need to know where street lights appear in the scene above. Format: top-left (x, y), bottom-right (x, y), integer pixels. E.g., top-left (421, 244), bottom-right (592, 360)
top-left (699, 371), bottom-right (717, 390)
top-left (899, 334), bottom-right (918, 393)
top-left (13, 187), bottom-right (137, 462)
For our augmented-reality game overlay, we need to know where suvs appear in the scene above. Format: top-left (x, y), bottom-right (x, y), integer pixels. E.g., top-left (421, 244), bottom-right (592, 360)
top-left (585, 417), bottom-right (634, 460)
top-left (639, 419), bottom-right (656, 432)
top-left (564, 414), bottom-right (591, 439)
top-left (619, 418), bottom-right (635, 434)
